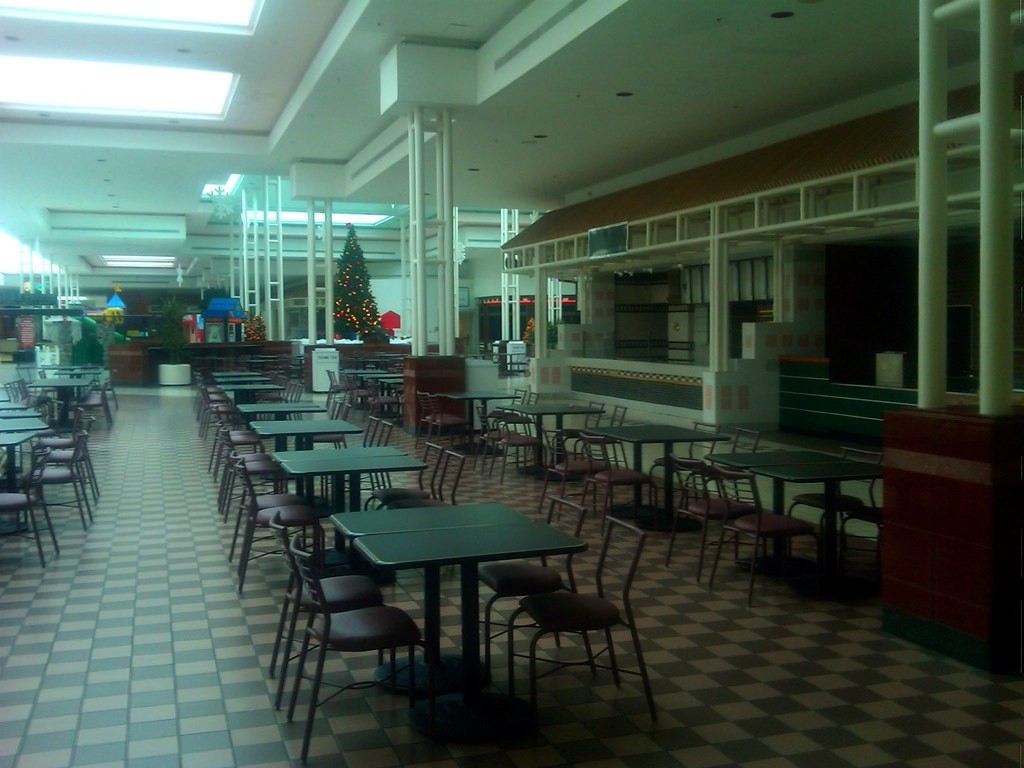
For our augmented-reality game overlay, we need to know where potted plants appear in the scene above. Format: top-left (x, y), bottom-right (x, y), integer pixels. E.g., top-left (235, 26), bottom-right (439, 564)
top-left (72, 315), bottom-right (112, 392)
top-left (152, 294), bottom-right (192, 386)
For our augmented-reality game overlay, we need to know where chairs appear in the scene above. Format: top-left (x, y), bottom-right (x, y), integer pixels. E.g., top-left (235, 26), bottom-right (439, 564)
top-left (506, 517), bottom-right (659, 728)
top-left (536, 425), bottom-right (607, 521)
top-left (664, 454), bottom-right (750, 584)
top-left (472, 405), bottom-right (527, 475)
top-left (552, 401), bottom-right (605, 461)
top-left (288, 533), bottom-right (436, 765)
top-left (186, 350), bottom-right (405, 442)
top-left (488, 422), bottom-right (542, 485)
top-left (426, 394), bottom-right (470, 449)
top-left (573, 404), bottom-right (628, 470)
top-left (488, 388), bottom-right (527, 431)
top-left (707, 464), bottom-right (823, 610)
top-left (787, 445), bottom-right (883, 567)
top-left (838, 508), bottom-right (884, 578)
top-left (699, 426), bottom-right (762, 501)
top-left (268, 511), bottom-right (398, 711)
top-left (577, 431), bottom-right (660, 538)
top-left (648, 423), bottom-right (722, 512)
top-left (497, 392), bottom-right (539, 451)
top-left (414, 391), bottom-right (455, 449)
top-left (213, 442), bottom-right (467, 595)
top-left (0, 366), bottom-right (119, 570)
top-left (477, 494), bottom-right (598, 680)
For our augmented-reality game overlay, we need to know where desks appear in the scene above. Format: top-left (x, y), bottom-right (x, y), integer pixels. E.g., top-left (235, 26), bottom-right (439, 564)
top-left (279, 455), bottom-right (429, 550)
top-left (211, 371), bottom-right (262, 384)
top-left (272, 447), bottom-right (408, 549)
top-left (352, 520), bottom-right (589, 747)
top-left (749, 460), bottom-right (883, 603)
top-left (240, 405), bottom-right (328, 452)
top-left (0, 401), bottom-right (29, 411)
top-left (214, 377), bottom-right (273, 385)
top-left (434, 390), bottom-right (732, 535)
top-left (216, 383), bottom-right (277, 422)
top-left (54, 371), bottom-right (101, 401)
top-left (704, 451), bottom-right (853, 580)
top-left (220, 386), bottom-right (286, 425)
top-left (35, 378), bottom-right (87, 426)
top-left (0, 409), bottom-right (42, 483)
top-left (326, 501), bottom-right (534, 701)
top-left (236, 402), bottom-right (320, 452)
top-left (0, 433), bottom-right (39, 537)
top-left (28, 382), bottom-right (89, 433)
top-left (254, 423), bottom-right (364, 518)
top-left (0, 417), bottom-right (49, 491)
top-left (38, 365), bottom-right (96, 378)
top-left (248, 419), bottom-right (345, 506)
